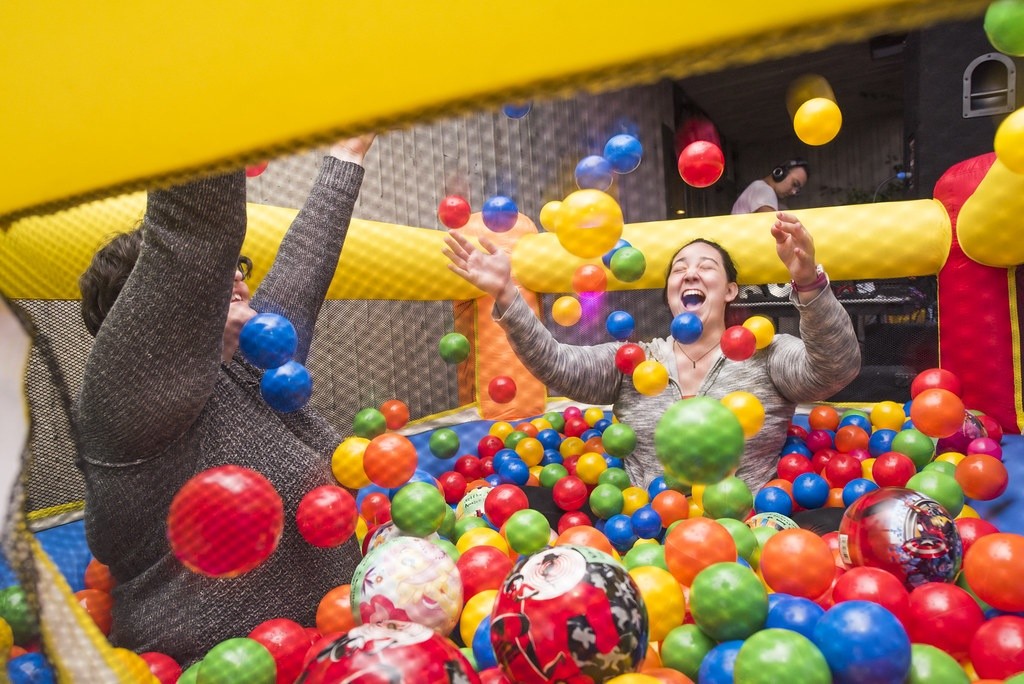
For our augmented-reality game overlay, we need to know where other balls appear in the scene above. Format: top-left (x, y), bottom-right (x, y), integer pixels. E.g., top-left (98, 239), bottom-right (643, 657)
top-left (0, 1), bottom-right (1023, 683)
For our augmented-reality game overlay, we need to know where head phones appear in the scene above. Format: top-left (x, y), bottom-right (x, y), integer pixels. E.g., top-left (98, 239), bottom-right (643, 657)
top-left (772, 159), bottom-right (808, 182)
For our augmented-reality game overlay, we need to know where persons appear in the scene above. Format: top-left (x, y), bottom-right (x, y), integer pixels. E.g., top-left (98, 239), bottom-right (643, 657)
top-left (731, 159), bottom-right (811, 216)
top-left (441, 211), bottom-right (862, 539)
top-left (74, 130), bottom-right (378, 675)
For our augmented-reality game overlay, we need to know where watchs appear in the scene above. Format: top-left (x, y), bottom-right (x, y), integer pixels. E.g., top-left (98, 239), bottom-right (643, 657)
top-left (791, 263), bottom-right (828, 292)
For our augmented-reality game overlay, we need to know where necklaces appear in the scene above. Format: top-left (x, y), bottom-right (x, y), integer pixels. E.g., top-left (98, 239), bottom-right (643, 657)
top-left (676, 341), bottom-right (721, 369)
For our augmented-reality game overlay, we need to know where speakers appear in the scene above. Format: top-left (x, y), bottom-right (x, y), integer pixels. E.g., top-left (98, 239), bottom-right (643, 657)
top-left (897, 16), bottom-right (1024, 202)
